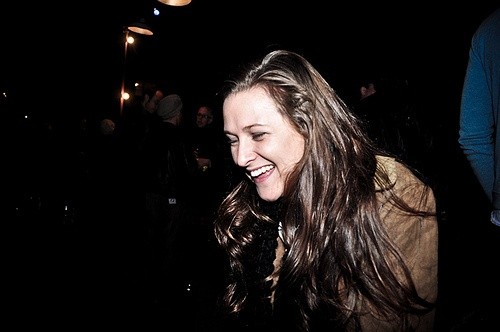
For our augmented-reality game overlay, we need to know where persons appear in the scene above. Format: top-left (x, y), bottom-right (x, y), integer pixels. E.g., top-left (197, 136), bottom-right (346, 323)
top-left (458, 15), bottom-right (500, 229)
top-left (214, 50), bottom-right (439, 332)
top-left (93, 73), bottom-right (427, 219)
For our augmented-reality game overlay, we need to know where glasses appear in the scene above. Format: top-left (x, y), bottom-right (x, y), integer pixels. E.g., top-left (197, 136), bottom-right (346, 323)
top-left (197, 113), bottom-right (211, 119)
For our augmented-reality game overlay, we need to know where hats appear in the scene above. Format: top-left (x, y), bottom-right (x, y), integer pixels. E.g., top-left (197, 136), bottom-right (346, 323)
top-left (156, 94), bottom-right (183, 119)
